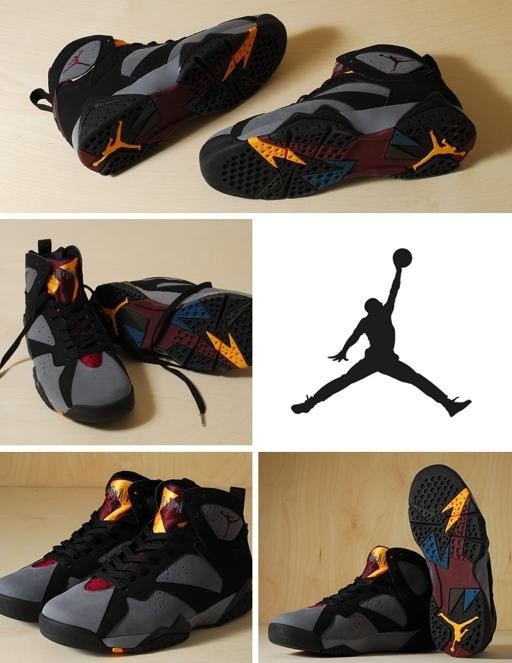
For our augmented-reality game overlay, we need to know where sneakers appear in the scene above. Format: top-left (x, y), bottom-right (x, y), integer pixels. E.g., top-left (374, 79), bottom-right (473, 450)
top-left (87, 275), bottom-right (252, 425)
top-left (0, 237), bottom-right (136, 426)
top-left (0, 469), bottom-right (195, 624)
top-left (291, 394), bottom-right (317, 414)
top-left (442, 395), bottom-right (472, 417)
top-left (29, 11), bottom-right (290, 177)
top-left (267, 545), bottom-right (442, 655)
top-left (37, 477), bottom-right (253, 658)
top-left (199, 43), bottom-right (480, 201)
top-left (406, 463), bottom-right (499, 659)
